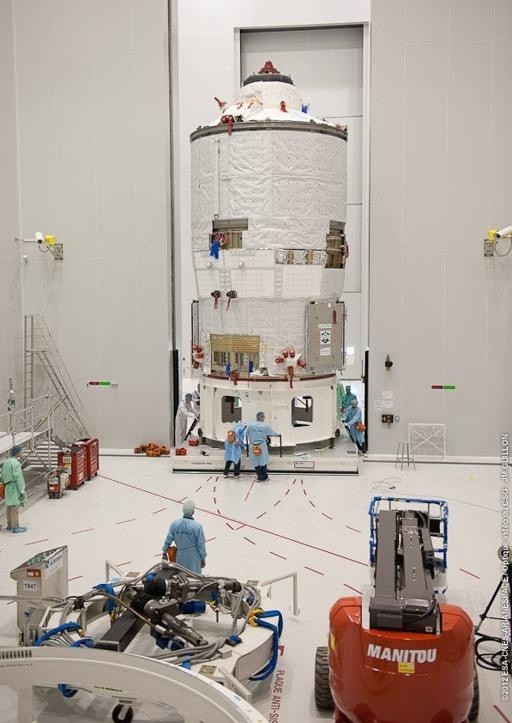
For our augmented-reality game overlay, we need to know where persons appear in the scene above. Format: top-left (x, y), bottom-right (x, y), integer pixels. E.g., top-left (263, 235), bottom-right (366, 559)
top-left (192, 384), bottom-right (200, 412)
top-left (245, 411), bottom-right (276, 482)
top-left (175, 393), bottom-right (199, 449)
top-left (1, 446), bottom-right (27, 533)
top-left (162, 500), bottom-right (206, 574)
top-left (223, 424), bottom-right (247, 478)
top-left (337, 383), bottom-right (366, 456)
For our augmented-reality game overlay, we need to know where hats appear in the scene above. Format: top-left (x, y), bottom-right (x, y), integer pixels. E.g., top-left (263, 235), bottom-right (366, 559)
top-left (182, 499), bottom-right (195, 516)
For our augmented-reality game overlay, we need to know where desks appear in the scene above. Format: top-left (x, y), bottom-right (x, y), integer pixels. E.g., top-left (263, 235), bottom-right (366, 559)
top-left (0, 431), bottom-right (47, 469)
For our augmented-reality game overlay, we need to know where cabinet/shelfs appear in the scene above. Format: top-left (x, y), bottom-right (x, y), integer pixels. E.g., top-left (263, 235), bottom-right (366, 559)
top-left (57, 436), bottom-right (99, 490)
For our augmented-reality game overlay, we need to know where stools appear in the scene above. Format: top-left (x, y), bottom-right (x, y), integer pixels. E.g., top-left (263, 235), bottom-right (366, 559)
top-left (395, 441), bottom-right (417, 471)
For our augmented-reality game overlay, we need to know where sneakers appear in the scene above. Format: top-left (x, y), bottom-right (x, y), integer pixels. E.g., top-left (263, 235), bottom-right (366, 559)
top-left (224, 473), bottom-right (244, 478)
top-left (6, 526), bottom-right (28, 533)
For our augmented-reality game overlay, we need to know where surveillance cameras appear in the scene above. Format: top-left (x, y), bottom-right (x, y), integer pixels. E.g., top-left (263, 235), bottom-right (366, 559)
top-left (495, 226), bottom-right (512, 239)
top-left (35, 233), bottom-right (43, 244)
